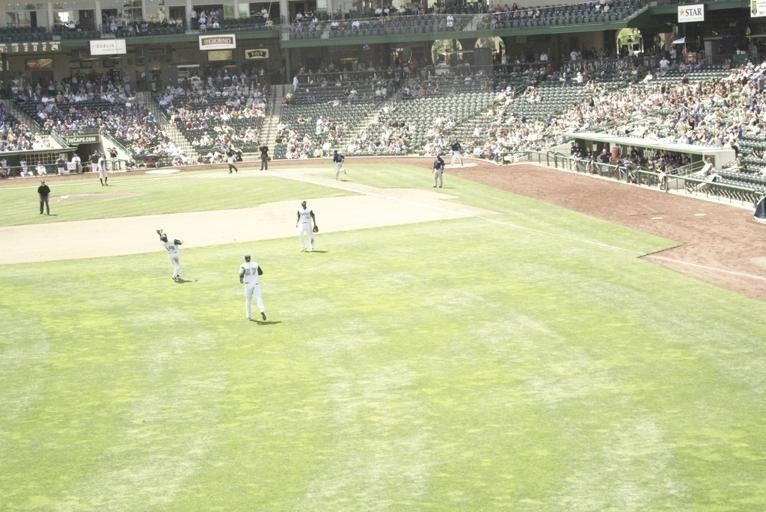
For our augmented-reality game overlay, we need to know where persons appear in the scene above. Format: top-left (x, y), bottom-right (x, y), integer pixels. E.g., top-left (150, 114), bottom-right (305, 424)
top-left (156, 227), bottom-right (184, 281)
top-left (62, 10), bottom-right (221, 37)
top-left (333, 150), bottom-right (348, 181)
top-left (226, 148), bottom-right (238, 173)
top-left (274, 59), bottom-right (475, 159)
top-left (1, 68), bottom-right (191, 174)
top-left (294, 201), bottom-right (318, 252)
top-left (627, 41), bottom-right (765, 187)
top-left (290, 1), bottom-right (540, 33)
top-left (461, 49), bottom-right (648, 166)
top-left (180, 66), bottom-right (267, 164)
top-left (34, 178), bottom-right (49, 214)
top-left (451, 140), bottom-right (464, 167)
top-left (595, 2), bottom-right (610, 14)
top-left (97, 152), bottom-right (112, 188)
top-left (237, 253), bottom-right (268, 322)
top-left (260, 9), bottom-right (270, 23)
top-left (432, 155), bottom-right (445, 187)
top-left (259, 143), bottom-right (268, 171)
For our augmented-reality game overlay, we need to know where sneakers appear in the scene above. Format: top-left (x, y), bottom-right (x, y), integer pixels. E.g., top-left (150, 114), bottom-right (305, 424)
top-left (172, 273), bottom-right (182, 282)
top-left (299, 246), bottom-right (316, 252)
top-left (261, 311), bottom-right (267, 321)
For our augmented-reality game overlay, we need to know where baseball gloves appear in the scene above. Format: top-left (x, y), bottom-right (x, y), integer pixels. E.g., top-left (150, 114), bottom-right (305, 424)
top-left (313, 226), bottom-right (318, 232)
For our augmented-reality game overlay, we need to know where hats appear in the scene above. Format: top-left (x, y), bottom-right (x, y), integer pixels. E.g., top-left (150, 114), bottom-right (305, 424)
top-left (160, 232), bottom-right (168, 240)
top-left (245, 255), bottom-right (251, 262)
top-left (301, 200), bottom-right (307, 207)
